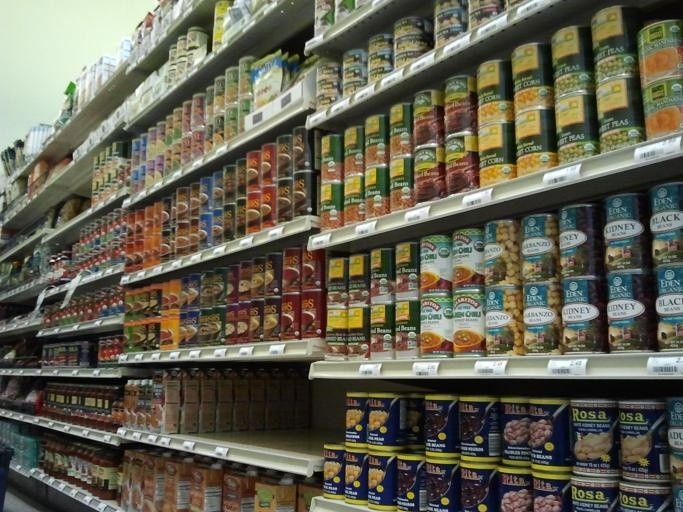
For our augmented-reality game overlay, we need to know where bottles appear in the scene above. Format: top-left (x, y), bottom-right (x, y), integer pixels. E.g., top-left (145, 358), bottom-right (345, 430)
top-left (41, 208), bottom-right (122, 505)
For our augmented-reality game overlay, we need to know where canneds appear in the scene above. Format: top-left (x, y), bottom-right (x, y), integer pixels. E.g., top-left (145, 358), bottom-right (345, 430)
top-left (48, 208), bottom-right (124, 280)
top-left (44, 381), bottom-right (123, 433)
top-left (43, 283), bottom-right (123, 327)
top-left (131, 55), bottom-right (257, 192)
top-left (91, 140), bottom-right (130, 207)
top-left (169, 2), bottom-right (232, 85)
top-left (123, 242), bottom-right (326, 350)
top-left (323, 390), bottom-right (682, 512)
top-left (42, 334), bottom-right (123, 367)
top-left (315, 0), bottom-right (682, 233)
top-left (323, 180), bottom-right (682, 358)
top-left (124, 124), bottom-right (314, 271)
top-left (44, 435), bottom-right (120, 499)
